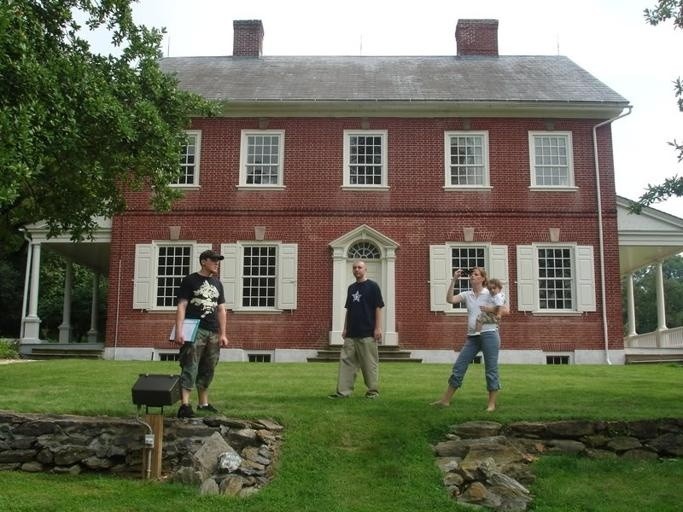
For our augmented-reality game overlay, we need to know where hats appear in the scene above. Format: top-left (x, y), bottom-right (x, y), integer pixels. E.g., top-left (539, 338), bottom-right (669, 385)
top-left (199, 250), bottom-right (225, 261)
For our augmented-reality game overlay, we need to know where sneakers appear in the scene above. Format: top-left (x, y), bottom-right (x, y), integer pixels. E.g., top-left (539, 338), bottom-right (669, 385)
top-left (366, 393), bottom-right (377, 399)
top-left (177, 404), bottom-right (218, 418)
top-left (328, 394), bottom-right (339, 399)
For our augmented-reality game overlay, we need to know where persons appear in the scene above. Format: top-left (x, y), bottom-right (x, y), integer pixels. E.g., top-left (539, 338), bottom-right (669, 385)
top-left (170, 248), bottom-right (229, 418)
top-left (426, 266), bottom-right (510, 411)
top-left (472, 278), bottom-right (504, 338)
top-left (326, 259), bottom-right (385, 399)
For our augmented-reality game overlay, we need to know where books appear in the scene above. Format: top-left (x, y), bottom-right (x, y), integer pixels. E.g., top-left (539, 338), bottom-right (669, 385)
top-left (169, 318), bottom-right (200, 343)
